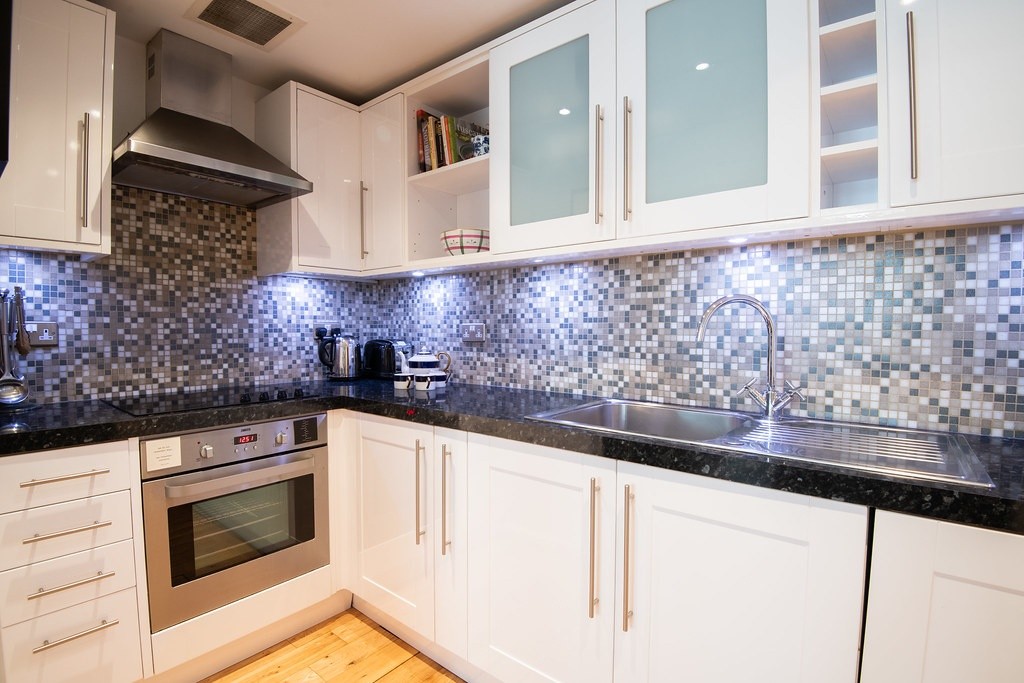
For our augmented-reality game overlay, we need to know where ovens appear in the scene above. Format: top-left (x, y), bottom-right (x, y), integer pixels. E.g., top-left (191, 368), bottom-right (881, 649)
top-left (139, 414), bottom-right (331, 635)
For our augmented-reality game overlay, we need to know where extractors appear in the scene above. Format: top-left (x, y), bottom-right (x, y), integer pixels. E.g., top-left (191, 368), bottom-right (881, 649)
top-left (112, 28), bottom-right (313, 211)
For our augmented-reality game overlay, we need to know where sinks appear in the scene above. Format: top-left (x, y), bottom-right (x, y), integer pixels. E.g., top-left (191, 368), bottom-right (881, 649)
top-left (523, 398), bottom-right (763, 451)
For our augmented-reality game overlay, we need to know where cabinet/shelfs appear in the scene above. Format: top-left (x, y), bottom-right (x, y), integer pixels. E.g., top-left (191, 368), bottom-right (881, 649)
top-left (1, 437), bottom-right (153, 683)
top-left (255, 0), bottom-right (1024, 282)
top-left (327, 410), bottom-right (1024, 681)
top-left (0, 0), bottom-right (116, 259)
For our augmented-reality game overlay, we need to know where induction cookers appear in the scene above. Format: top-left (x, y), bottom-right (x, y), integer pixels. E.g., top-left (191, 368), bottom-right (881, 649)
top-left (100, 384), bottom-right (320, 417)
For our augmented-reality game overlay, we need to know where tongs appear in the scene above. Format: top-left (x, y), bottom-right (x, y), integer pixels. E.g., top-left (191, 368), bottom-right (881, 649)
top-left (15, 294), bottom-right (31, 355)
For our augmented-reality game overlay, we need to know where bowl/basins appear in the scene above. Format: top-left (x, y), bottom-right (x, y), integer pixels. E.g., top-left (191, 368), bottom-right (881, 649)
top-left (439, 228), bottom-right (490, 256)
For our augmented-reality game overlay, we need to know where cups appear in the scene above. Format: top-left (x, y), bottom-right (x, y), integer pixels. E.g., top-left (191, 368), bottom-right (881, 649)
top-left (436, 373), bottom-right (452, 387)
top-left (415, 374), bottom-right (436, 390)
top-left (459, 135), bottom-right (489, 161)
top-left (393, 374), bottom-right (414, 389)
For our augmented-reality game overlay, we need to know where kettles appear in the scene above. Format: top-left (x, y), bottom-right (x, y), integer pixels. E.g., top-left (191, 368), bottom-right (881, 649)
top-left (318, 336), bottom-right (361, 382)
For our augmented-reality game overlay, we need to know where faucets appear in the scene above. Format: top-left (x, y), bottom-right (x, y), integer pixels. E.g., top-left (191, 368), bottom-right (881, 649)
top-left (695, 293), bottom-right (807, 419)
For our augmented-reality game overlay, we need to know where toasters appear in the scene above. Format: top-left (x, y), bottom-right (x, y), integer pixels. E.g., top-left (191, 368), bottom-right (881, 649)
top-left (363, 340), bottom-right (396, 379)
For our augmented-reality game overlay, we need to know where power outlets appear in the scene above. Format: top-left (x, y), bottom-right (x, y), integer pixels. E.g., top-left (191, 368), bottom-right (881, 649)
top-left (4, 320), bottom-right (58, 348)
top-left (460, 322), bottom-right (485, 342)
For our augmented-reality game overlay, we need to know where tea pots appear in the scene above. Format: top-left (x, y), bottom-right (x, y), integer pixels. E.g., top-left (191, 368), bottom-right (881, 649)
top-left (397, 346), bottom-right (452, 374)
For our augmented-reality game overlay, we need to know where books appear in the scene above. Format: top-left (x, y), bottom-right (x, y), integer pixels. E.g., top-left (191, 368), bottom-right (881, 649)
top-left (416, 109), bottom-right (488, 174)
